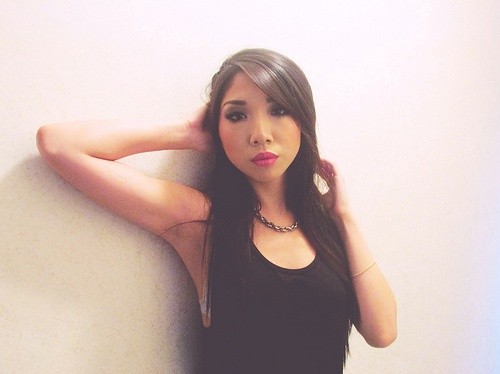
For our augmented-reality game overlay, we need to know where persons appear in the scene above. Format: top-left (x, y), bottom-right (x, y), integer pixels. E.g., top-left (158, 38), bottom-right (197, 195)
top-left (36, 48), bottom-right (397, 373)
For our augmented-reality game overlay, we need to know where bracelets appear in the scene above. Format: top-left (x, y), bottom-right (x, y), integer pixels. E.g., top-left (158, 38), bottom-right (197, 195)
top-left (351, 257), bottom-right (376, 278)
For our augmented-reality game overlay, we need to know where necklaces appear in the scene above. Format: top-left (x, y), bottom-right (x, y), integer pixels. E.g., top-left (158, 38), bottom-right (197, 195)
top-left (254, 209), bottom-right (301, 232)
